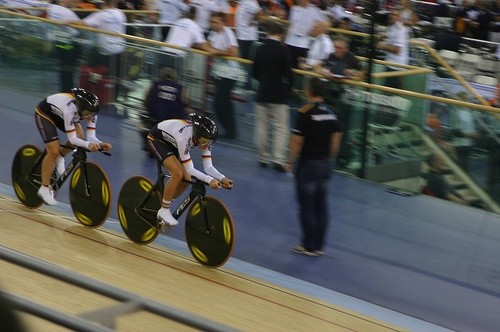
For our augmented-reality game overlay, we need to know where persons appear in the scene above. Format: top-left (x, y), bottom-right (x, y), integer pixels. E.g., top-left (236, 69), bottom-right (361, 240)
top-left (147, 115), bottom-right (233, 226)
top-left (283, 76), bottom-right (346, 256)
top-left (34, 87), bottom-right (112, 206)
top-left (46, 1), bottom-right (495, 175)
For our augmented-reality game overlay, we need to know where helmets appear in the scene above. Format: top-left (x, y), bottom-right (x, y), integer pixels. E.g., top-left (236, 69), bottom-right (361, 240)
top-left (189, 112), bottom-right (218, 143)
top-left (70, 87), bottom-right (100, 114)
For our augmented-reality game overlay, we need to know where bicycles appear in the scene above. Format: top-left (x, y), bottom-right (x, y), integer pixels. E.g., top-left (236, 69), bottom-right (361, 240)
top-left (11, 144), bottom-right (112, 228)
top-left (118, 152), bottom-right (235, 268)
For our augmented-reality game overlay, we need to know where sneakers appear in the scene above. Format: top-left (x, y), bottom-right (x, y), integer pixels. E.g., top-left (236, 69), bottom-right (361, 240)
top-left (55, 154), bottom-right (66, 176)
top-left (157, 207), bottom-right (180, 226)
top-left (37, 185), bottom-right (57, 206)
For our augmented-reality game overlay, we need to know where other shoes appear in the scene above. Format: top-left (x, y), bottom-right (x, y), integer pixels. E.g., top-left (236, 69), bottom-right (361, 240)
top-left (292, 244), bottom-right (324, 256)
top-left (274, 162), bottom-right (288, 171)
top-left (259, 162), bottom-right (267, 167)
